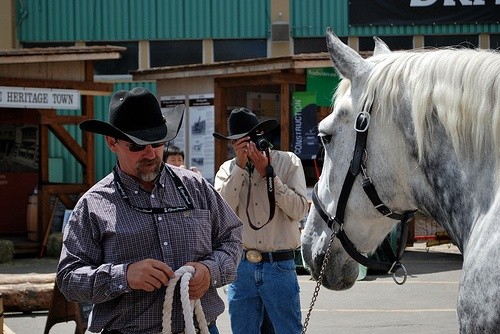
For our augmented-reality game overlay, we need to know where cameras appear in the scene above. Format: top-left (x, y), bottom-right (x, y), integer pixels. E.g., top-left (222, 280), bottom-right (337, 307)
top-left (247, 130), bottom-right (275, 151)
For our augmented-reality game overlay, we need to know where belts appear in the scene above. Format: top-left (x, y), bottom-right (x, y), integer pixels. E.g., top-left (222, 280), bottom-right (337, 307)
top-left (246, 250), bottom-right (294, 263)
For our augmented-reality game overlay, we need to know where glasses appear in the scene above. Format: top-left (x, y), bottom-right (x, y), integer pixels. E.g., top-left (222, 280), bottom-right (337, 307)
top-left (117, 139), bottom-right (164, 151)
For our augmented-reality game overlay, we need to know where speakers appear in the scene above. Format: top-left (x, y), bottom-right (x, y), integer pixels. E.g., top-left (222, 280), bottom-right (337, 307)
top-left (271, 22), bottom-right (290, 43)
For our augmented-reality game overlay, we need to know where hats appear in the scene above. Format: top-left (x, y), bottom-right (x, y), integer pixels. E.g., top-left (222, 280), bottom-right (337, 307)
top-left (79, 87), bottom-right (185, 145)
top-left (212, 108), bottom-right (278, 139)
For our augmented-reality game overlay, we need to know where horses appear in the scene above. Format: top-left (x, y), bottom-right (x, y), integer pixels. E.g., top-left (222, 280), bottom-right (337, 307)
top-left (300, 27), bottom-right (500, 334)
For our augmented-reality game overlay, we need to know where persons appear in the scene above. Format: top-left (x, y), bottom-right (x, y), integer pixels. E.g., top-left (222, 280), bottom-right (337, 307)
top-left (211, 107), bottom-right (309, 334)
top-left (56, 86), bottom-right (244, 334)
top-left (163, 146), bottom-right (201, 175)
